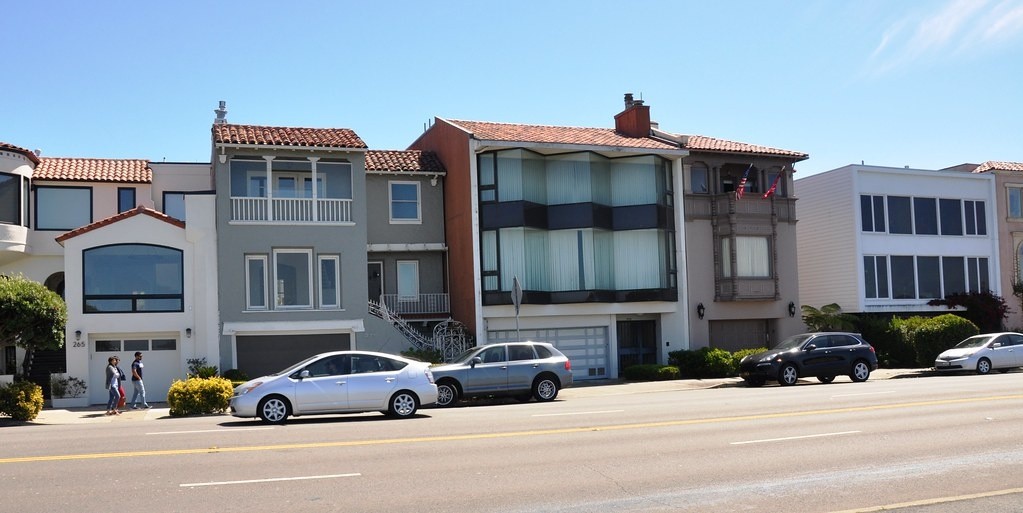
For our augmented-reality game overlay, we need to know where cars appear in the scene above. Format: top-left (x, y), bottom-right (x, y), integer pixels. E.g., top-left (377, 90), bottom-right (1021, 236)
top-left (934, 332), bottom-right (1023, 376)
top-left (229, 350), bottom-right (439, 425)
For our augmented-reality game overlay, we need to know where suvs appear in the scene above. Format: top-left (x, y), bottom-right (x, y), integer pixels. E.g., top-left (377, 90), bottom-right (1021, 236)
top-left (428, 342), bottom-right (574, 409)
top-left (737, 332), bottom-right (878, 387)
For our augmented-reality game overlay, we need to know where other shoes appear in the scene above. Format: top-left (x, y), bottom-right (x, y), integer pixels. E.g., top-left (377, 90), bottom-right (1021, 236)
top-left (141, 405), bottom-right (152, 409)
top-left (130, 407), bottom-right (141, 409)
top-left (106, 408), bottom-right (128, 415)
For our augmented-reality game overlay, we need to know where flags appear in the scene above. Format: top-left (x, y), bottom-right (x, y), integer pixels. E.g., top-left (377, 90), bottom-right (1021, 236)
top-left (735, 166), bottom-right (751, 201)
top-left (762, 172), bottom-right (781, 200)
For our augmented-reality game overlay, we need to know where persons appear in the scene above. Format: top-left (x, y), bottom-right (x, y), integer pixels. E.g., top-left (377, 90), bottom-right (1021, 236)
top-left (492, 353), bottom-right (499, 361)
top-left (129, 352), bottom-right (150, 411)
top-left (105, 355), bottom-right (127, 415)
top-left (311, 360), bottom-right (338, 376)
